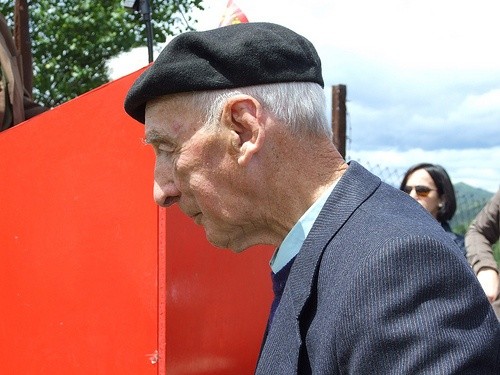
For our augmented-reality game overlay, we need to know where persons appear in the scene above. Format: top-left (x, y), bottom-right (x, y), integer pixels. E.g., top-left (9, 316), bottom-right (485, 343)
top-left (396, 164), bottom-right (467, 261)
top-left (464, 189), bottom-right (499, 304)
top-left (125, 21), bottom-right (499, 375)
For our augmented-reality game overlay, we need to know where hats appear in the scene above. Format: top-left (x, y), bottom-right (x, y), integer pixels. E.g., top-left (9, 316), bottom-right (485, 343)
top-left (124, 23), bottom-right (325, 124)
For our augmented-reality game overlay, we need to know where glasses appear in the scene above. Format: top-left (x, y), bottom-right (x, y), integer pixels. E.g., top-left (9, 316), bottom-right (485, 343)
top-left (402, 186), bottom-right (438, 197)
